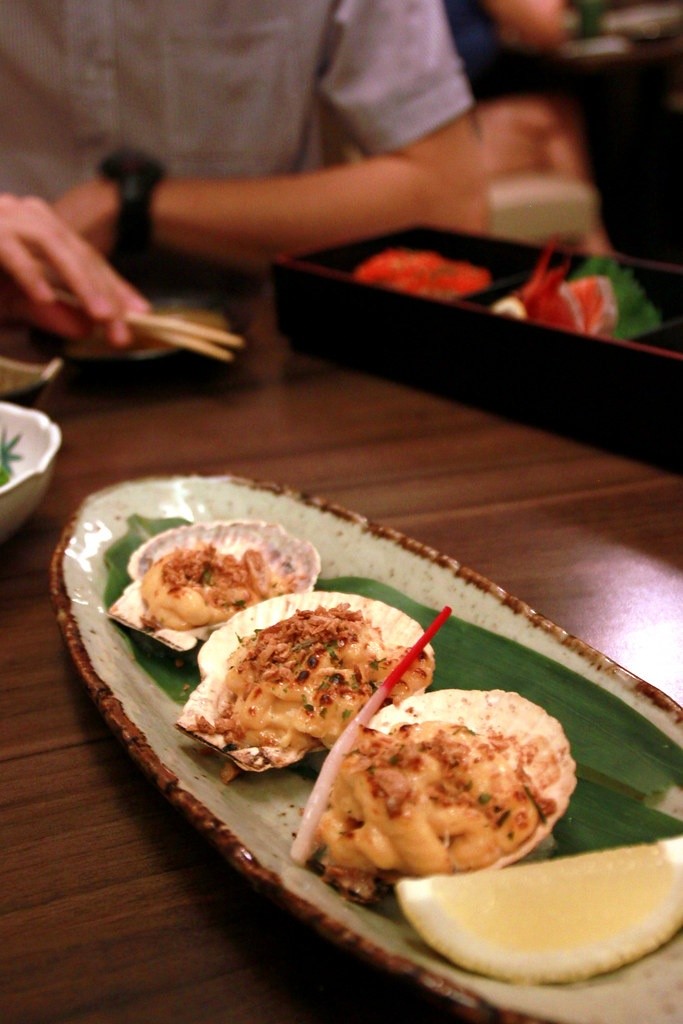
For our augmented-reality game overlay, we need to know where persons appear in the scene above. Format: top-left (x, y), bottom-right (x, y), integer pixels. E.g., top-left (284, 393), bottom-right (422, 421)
top-left (0, 0), bottom-right (683, 352)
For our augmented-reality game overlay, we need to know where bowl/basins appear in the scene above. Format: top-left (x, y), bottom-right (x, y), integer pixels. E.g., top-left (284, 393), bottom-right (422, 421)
top-left (0, 357), bottom-right (52, 411)
top-left (0, 400), bottom-right (63, 544)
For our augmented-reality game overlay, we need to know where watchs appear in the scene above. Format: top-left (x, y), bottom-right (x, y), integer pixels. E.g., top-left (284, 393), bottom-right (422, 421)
top-left (99, 152), bottom-right (167, 256)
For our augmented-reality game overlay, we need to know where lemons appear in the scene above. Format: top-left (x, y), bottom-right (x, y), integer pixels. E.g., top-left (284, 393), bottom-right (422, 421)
top-left (396, 834), bottom-right (683, 982)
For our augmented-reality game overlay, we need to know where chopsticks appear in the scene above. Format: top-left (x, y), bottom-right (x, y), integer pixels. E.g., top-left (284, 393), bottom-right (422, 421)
top-left (61, 295), bottom-right (244, 364)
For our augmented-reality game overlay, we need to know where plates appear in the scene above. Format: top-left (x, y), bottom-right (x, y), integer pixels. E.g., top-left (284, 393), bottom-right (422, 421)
top-left (36, 299), bottom-right (226, 361)
top-left (50, 472), bottom-right (683, 1024)
top-left (272, 220), bottom-right (683, 472)
